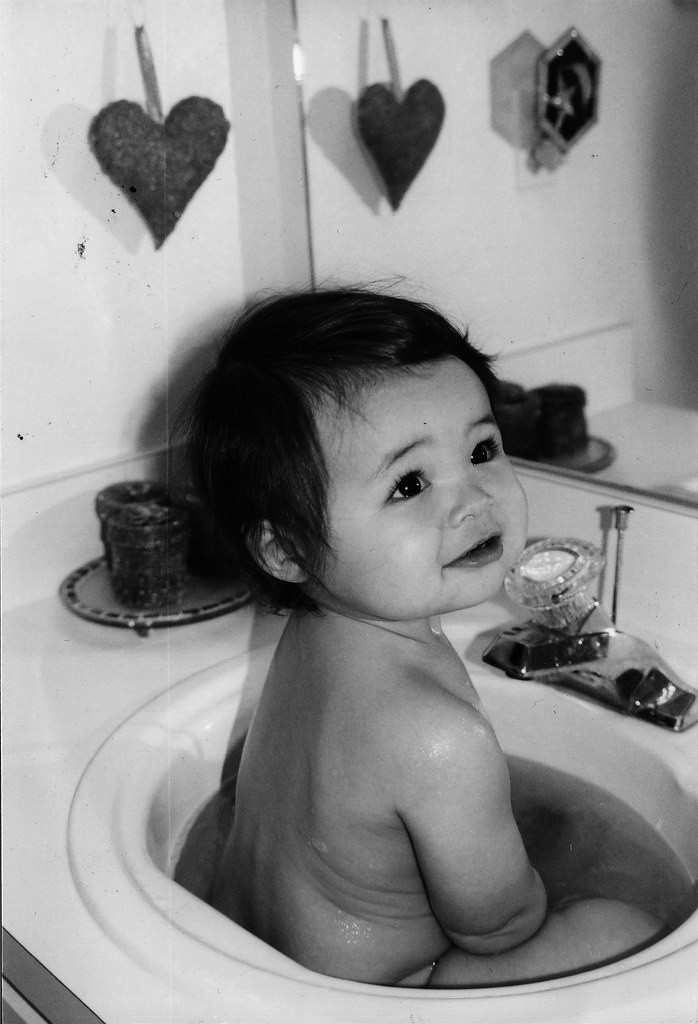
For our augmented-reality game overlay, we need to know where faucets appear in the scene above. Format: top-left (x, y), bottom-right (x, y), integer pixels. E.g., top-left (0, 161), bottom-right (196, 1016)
top-left (503, 624), bottom-right (643, 707)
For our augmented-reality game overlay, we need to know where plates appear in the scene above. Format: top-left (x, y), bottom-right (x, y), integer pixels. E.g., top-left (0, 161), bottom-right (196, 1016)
top-left (59, 551), bottom-right (262, 627)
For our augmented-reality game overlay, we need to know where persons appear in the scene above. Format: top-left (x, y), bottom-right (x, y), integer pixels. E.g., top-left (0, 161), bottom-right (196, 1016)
top-left (178, 284), bottom-right (660, 987)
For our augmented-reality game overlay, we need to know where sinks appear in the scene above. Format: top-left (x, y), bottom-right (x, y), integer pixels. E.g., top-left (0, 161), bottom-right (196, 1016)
top-left (65, 641), bottom-right (698, 1024)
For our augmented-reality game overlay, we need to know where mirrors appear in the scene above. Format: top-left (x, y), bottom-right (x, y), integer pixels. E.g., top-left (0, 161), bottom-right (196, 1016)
top-left (291, 1), bottom-right (698, 516)
